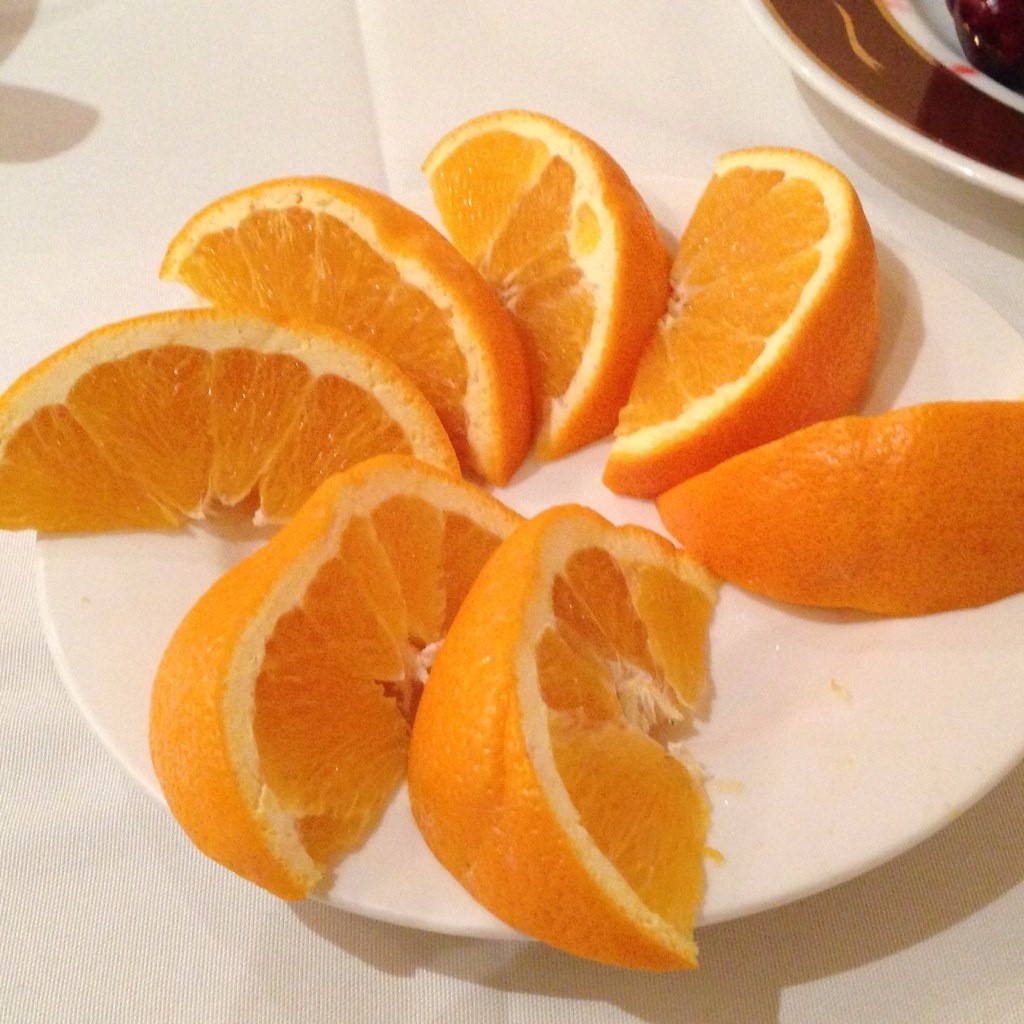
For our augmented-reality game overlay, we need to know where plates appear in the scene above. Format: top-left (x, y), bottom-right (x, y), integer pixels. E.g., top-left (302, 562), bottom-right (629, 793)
top-left (737, 0), bottom-right (1024, 203)
top-left (882, 0), bottom-right (1024, 113)
top-left (27, 170), bottom-right (1024, 943)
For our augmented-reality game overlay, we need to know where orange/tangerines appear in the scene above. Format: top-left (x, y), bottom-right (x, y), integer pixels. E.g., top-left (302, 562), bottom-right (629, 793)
top-left (0, 108), bottom-right (1023, 969)
